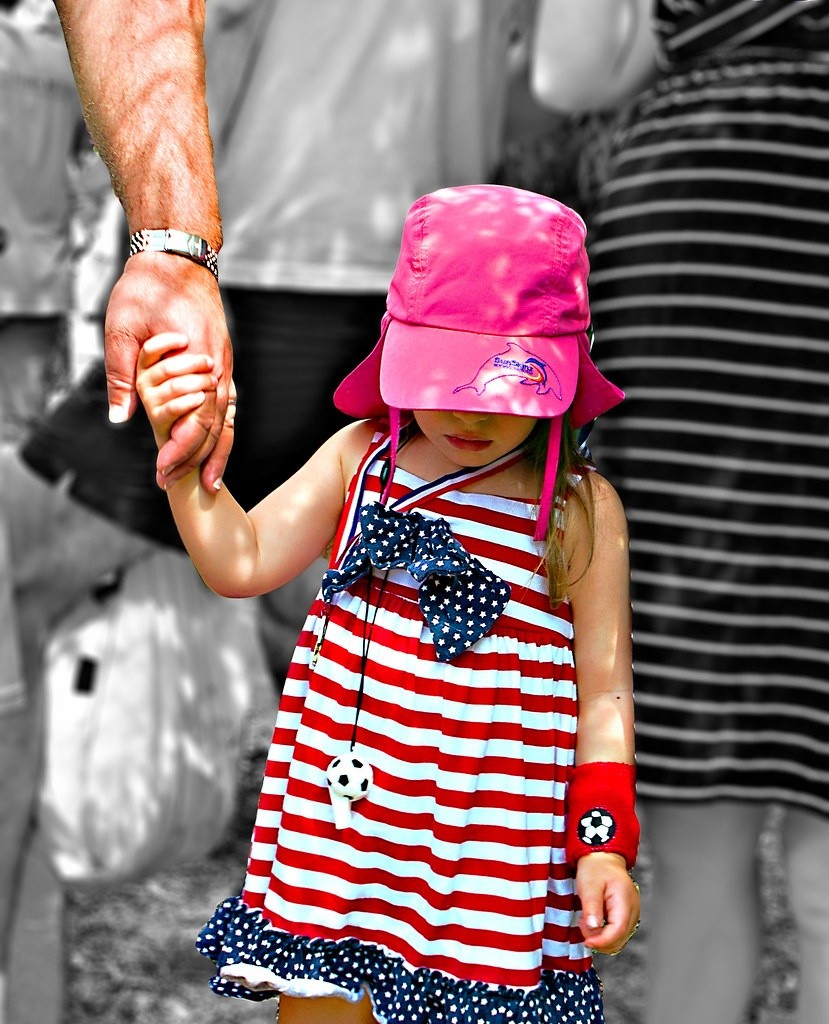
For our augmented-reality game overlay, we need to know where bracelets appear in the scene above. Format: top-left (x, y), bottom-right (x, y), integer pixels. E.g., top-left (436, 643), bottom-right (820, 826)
top-left (129, 228), bottom-right (218, 282)
top-left (567, 761), bottom-right (641, 871)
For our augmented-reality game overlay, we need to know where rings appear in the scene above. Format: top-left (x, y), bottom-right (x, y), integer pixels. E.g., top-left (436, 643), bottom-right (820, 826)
top-left (228, 399), bottom-right (236, 405)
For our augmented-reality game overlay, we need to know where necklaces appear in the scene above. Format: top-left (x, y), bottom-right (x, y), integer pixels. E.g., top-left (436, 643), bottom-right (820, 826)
top-left (327, 427), bottom-right (420, 801)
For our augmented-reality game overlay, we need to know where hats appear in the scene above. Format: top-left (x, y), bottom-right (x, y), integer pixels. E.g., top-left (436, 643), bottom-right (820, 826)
top-left (332, 184), bottom-right (625, 429)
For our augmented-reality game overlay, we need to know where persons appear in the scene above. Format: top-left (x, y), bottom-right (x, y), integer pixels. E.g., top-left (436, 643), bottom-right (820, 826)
top-left (17, 0), bottom-right (512, 556)
top-left (53, 0), bottom-right (238, 497)
top-left (532, 0), bottom-right (829, 1024)
top-left (135, 184), bottom-right (641, 1024)
